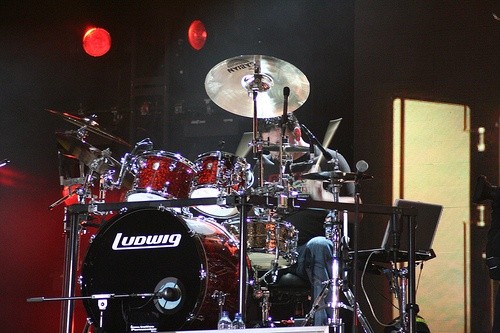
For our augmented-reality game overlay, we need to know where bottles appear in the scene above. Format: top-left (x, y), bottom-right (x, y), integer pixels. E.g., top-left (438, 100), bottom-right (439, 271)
top-left (218, 311), bottom-right (232, 330)
top-left (233, 313), bottom-right (245, 329)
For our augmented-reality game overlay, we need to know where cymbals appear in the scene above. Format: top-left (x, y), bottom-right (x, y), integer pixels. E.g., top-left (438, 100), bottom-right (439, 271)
top-left (265, 140), bottom-right (308, 155)
top-left (298, 170), bottom-right (374, 185)
top-left (44, 107), bottom-right (126, 144)
top-left (203, 54), bottom-right (311, 119)
top-left (53, 129), bottom-right (132, 190)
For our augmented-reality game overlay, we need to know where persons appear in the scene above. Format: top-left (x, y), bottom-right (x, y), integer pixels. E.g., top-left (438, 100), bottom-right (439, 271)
top-left (252, 113), bottom-right (362, 326)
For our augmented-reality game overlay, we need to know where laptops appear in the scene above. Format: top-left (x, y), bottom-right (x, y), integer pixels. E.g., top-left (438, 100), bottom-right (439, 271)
top-left (347, 199), bottom-right (443, 254)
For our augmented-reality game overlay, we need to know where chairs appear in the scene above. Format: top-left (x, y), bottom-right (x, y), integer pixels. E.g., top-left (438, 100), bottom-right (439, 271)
top-left (350, 200), bottom-right (442, 333)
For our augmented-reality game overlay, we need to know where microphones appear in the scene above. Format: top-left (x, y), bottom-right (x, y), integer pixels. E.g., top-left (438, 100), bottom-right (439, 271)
top-left (300, 125), bottom-right (332, 162)
top-left (162, 287), bottom-right (180, 301)
top-left (353, 160), bottom-right (369, 185)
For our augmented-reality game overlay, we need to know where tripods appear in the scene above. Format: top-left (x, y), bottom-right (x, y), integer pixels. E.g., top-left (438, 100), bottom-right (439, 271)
top-left (300, 185), bottom-right (374, 333)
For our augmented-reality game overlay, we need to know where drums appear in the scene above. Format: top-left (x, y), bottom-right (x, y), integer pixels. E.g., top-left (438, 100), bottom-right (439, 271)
top-left (80, 206), bottom-right (250, 333)
top-left (189, 151), bottom-right (253, 219)
top-left (124, 148), bottom-right (198, 215)
top-left (223, 218), bottom-right (298, 273)
